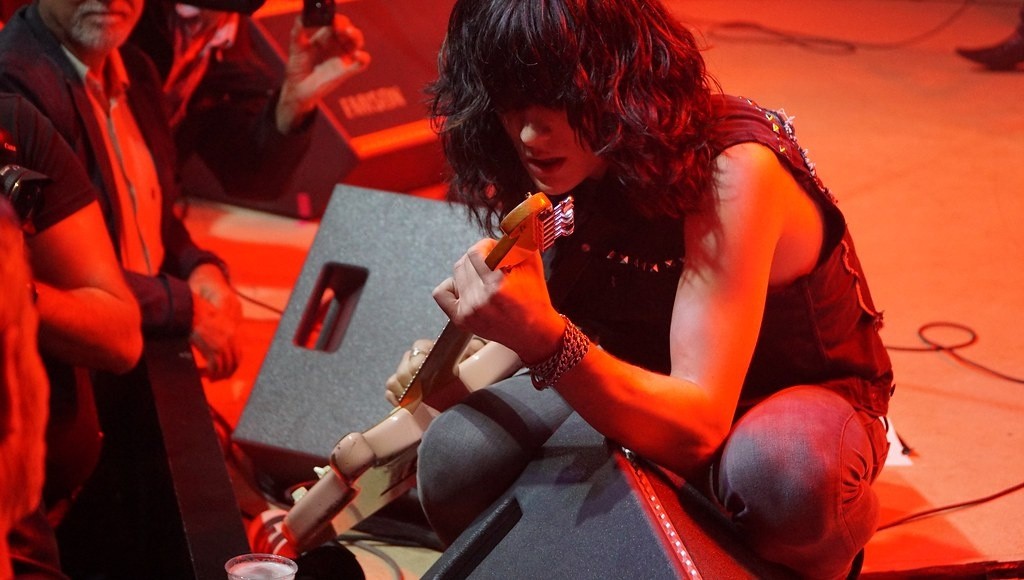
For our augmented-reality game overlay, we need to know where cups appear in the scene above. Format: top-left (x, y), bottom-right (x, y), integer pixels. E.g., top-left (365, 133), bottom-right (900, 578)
top-left (225, 553), bottom-right (298, 580)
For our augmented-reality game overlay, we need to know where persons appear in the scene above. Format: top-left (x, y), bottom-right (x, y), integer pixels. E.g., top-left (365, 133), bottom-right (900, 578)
top-left (385, 0), bottom-right (895, 580)
top-left (134, 0), bottom-right (375, 204)
top-left (1, 94), bottom-right (148, 580)
top-left (0, 0), bottom-right (252, 579)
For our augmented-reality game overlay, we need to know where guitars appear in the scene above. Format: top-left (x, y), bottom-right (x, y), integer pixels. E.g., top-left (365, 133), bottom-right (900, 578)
top-left (282, 192), bottom-right (573, 556)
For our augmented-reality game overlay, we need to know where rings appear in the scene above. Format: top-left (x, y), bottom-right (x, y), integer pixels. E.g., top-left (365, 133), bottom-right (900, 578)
top-left (411, 347), bottom-right (420, 356)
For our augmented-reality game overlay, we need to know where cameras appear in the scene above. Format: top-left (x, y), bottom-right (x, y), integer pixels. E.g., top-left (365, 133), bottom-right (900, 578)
top-left (0, 156), bottom-right (53, 222)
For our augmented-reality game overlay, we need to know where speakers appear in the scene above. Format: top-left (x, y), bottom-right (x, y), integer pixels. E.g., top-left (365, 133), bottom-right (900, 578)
top-left (170, 0), bottom-right (797, 579)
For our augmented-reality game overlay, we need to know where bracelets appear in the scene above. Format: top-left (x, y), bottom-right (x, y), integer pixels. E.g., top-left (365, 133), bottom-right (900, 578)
top-left (519, 314), bottom-right (592, 391)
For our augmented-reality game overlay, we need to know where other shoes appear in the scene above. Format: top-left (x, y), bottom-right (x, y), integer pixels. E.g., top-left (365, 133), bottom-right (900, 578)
top-left (955, 29), bottom-right (1024, 75)
